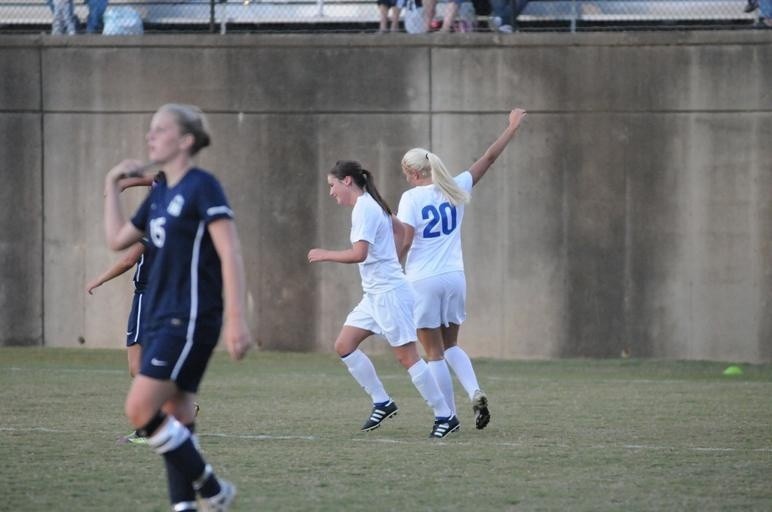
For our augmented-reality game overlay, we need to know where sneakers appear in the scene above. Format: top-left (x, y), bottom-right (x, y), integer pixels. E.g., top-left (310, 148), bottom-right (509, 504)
top-left (472, 390), bottom-right (490, 430)
top-left (116, 431), bottom-right (149, 444)
top-left (360, 396), bottom-right (399, 432)
top-left (193, 401), bottom-right (200, 417)
top-left (428, 410), bottom-right (460, 438)
top-left (200, 481), bottom-right (235, 512)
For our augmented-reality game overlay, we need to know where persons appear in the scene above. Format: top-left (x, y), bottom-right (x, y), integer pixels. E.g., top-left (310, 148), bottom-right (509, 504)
top-left (420, 0), bottom-right (464, 35)
top-left (374, 1), bottom-right (407, 34)
top-left (308, 159), bottom-right (462, 440)
top-left (86, 172), bottom-right (161, 444)
top-left (396, 107), bottom-right (527, 430)
top-left (48, 0), bottom-right (79, 35)
top-left (486, 1), bottom-right (529, 35)
top-left (102, 100), bottom-right (259, 512)
top-left (84, 0), bottom-right (108, 35)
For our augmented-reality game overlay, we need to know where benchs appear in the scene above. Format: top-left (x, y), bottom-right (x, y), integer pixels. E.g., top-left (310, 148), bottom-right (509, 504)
top-left (0, 0), bottom-right (761, 35)
